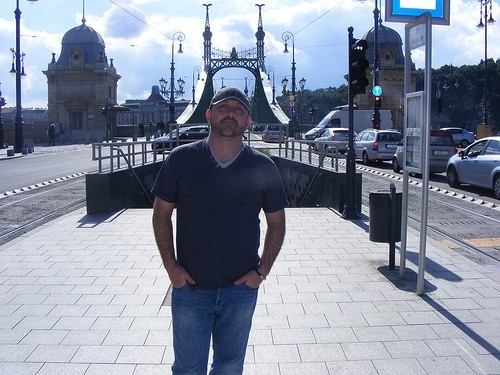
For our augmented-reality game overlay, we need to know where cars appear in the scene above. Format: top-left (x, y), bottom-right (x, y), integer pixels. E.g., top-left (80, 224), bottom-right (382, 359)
top-left (151, 124), bottom-right (212, 153)
top-left (446, 136), bottom-right (500, 200)
top-left (441, 127), bottom-right (475, 148)
top-left (261, 124), bottom-right (286, 143)
top-left (352, 128), bottom-right (405, 165)
top-left (391, 129), bottom-right (458, 177)
top-left (313, 128), bottom-right (357, 155)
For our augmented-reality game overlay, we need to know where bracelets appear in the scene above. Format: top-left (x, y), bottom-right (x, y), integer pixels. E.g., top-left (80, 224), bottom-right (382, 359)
top-left (254, 267), bottom-right (266, 280)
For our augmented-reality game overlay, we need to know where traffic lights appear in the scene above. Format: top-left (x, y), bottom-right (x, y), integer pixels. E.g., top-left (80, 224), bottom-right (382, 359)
top-left (375, 97), bottom-right (381, 108)
top-left (349, 39), bottom-right (370, 95)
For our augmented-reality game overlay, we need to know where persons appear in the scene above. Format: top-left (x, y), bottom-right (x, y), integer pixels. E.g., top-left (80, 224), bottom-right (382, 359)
top-left (150, 85), bottom-right (289, 375)
top-left (138, 122), bottom-right (145, 137)
top-left (48, 123), bottom-right (56, 146)
top-left (148, 120), bottom-right (171, 138)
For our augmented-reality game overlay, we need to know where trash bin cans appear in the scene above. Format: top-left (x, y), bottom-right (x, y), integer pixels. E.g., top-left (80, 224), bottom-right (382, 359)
top-left (369, 189), bottom-right (402, 243)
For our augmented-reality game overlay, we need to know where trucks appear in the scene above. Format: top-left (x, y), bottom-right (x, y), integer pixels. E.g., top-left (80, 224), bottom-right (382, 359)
top-left (304, 109), bottom-right (393, 150)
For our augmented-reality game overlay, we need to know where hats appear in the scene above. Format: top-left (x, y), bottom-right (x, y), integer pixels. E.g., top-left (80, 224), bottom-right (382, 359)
top-left (209, 87), bottom-right (251, 111)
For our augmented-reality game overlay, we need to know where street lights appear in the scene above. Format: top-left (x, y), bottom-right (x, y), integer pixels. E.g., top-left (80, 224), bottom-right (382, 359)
top-left (160, 31), bottom-right (186, 130)
top-left (444, 63), bottom-right (460, 128)
top-left (477, 0), bottom-right (496, 137)
top-left (9, 0), bottom-right (37, 152)
top-left (280, 31), bottom-right (307, 138)
